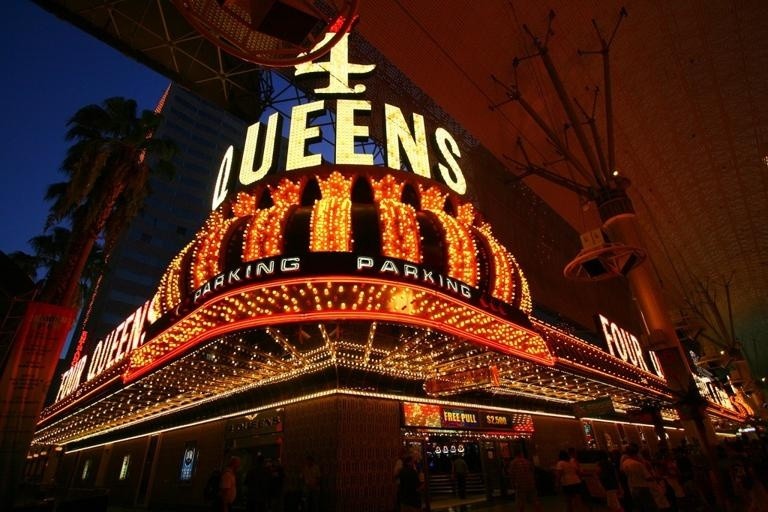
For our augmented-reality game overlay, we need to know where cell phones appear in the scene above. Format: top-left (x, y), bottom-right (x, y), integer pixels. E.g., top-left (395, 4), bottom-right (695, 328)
top-left (180, 444), bottom-right (197, 481)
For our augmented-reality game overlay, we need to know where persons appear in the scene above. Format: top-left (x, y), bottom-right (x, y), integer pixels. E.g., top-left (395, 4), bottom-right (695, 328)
top-left (204, 453), bottom-right (328, 512)
top-left (392, 451), bottom-right (545, 512)
top-left (553, 431), bottom-right (768, 511)
